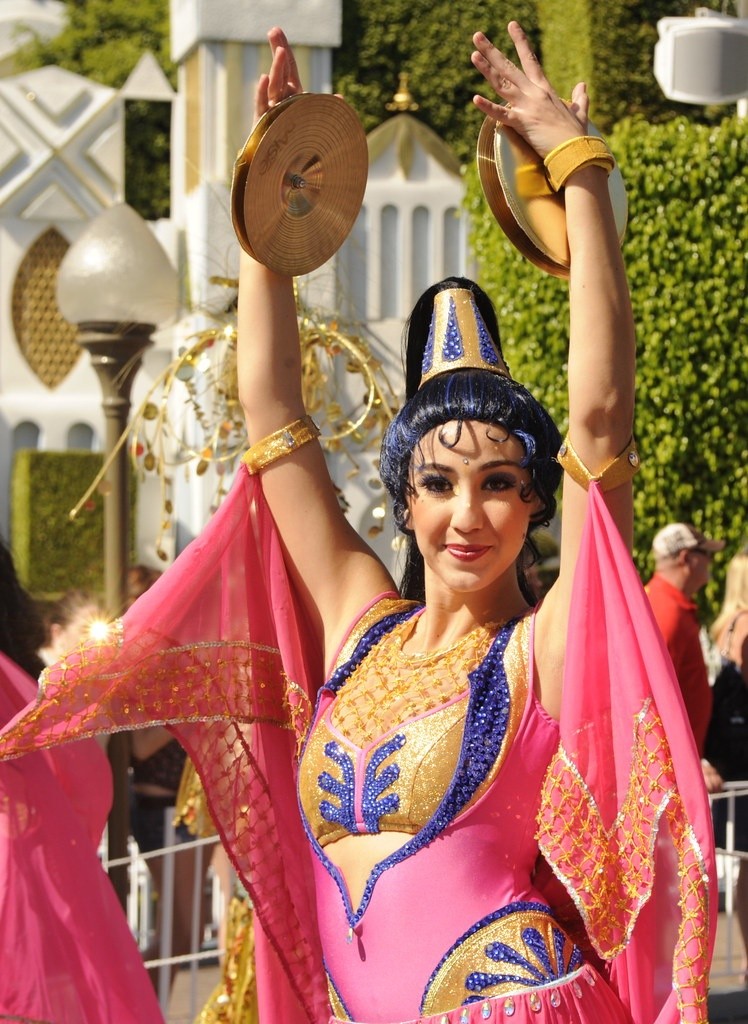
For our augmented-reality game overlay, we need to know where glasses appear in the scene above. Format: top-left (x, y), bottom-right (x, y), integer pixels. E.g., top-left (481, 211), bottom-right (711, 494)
top-left (690, 547), bottom-right (714, 559)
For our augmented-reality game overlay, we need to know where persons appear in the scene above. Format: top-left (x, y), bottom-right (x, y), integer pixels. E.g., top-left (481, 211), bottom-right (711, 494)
top-left (644, 522), bottom-right (724, 755)
top-left (0, 20), bottom-right (718, 1023)
top-left (1, 546), bottom-right (161, 1022)
top-left (700, 543), bottom-right (748, 957)
top-left (122, 562), bottom-right (195, 866)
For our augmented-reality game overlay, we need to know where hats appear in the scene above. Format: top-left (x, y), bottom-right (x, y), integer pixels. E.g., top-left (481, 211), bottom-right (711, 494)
top-left (652, 522), bottom-right (726, 560)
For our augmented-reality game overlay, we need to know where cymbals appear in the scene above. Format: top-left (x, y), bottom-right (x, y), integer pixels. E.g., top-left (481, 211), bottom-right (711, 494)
top-left (229, 91), bottom-right (332, 266)
top-left (243, 92), bottom-right (369, 278)
top-left (473, 95), bottom-right (629, 281)
top-left (492, 100), bottom-right (629, 274)
